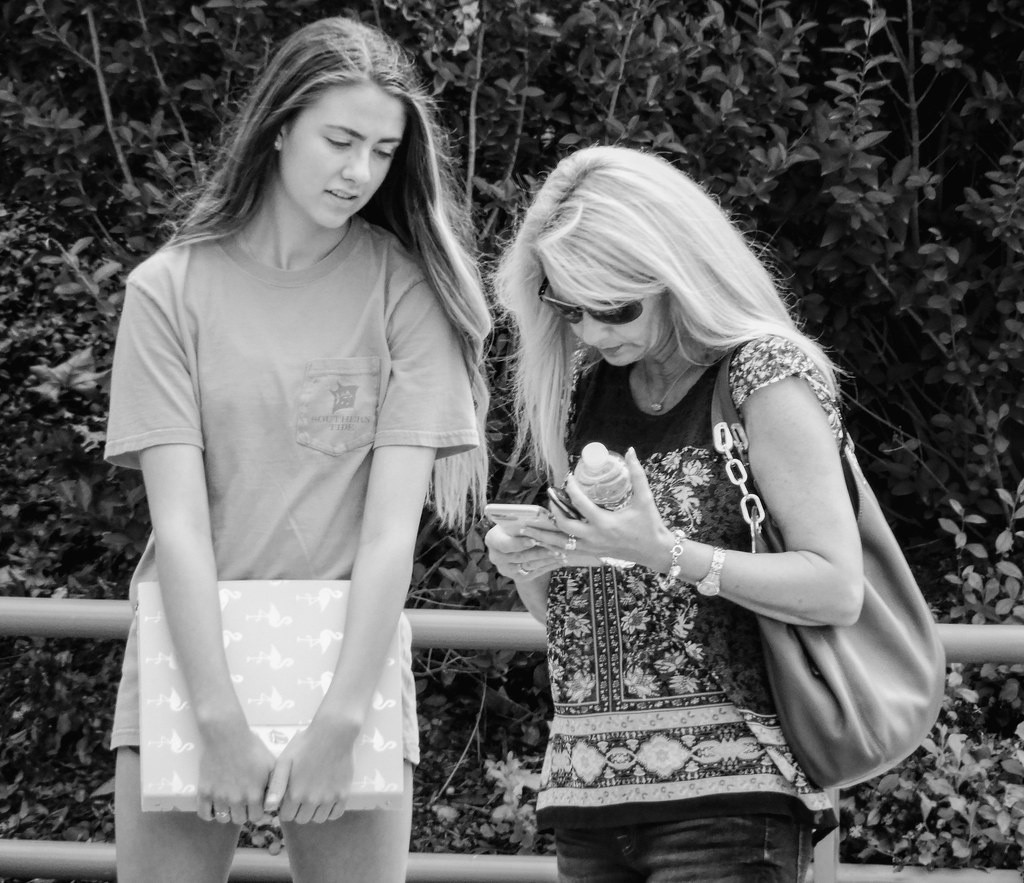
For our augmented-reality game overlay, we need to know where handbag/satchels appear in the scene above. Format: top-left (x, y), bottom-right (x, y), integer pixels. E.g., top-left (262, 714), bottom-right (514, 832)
top-left (711, 344), bottom-right (943, 791)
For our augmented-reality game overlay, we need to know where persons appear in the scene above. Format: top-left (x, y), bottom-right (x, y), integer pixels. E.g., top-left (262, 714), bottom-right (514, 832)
top-left (484, 141), bottom-right (871, 882)
top-left (99, 12), bottom-right (496, 883)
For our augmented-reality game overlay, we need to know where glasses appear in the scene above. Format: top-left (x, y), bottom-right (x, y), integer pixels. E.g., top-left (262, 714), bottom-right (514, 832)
top-left (537, 277), bottom-right (643, 324)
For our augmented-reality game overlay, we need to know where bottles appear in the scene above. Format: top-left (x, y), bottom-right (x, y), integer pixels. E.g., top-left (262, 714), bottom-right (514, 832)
top-left (574, 441), bottom-right (636, 568)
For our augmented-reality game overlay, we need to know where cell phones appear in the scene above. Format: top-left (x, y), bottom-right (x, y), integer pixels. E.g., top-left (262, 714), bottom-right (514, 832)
top-left (483, 502), bottom-right (551, 525)
top-left (548, 487), bottom-right (583, 522)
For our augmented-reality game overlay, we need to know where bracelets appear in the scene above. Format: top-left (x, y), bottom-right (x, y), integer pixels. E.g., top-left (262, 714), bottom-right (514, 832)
top-left (653, 530), bottom-right (688, 591)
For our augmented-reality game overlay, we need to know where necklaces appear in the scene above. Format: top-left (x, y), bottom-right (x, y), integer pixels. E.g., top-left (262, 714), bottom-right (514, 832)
top-left (640, 341), bottom-right (713, 411)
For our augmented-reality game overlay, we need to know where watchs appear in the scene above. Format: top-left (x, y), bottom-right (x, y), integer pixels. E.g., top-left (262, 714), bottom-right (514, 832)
top-left (695, 548), bottom-right (725, 596)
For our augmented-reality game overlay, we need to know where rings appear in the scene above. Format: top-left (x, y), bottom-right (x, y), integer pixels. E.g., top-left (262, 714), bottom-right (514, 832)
top-left (516, 562), bottom-right (530, 576)
top-left (214, 811), bottom-right (229, 817)
top-left (564, 535), bottom-right (577, 551)
